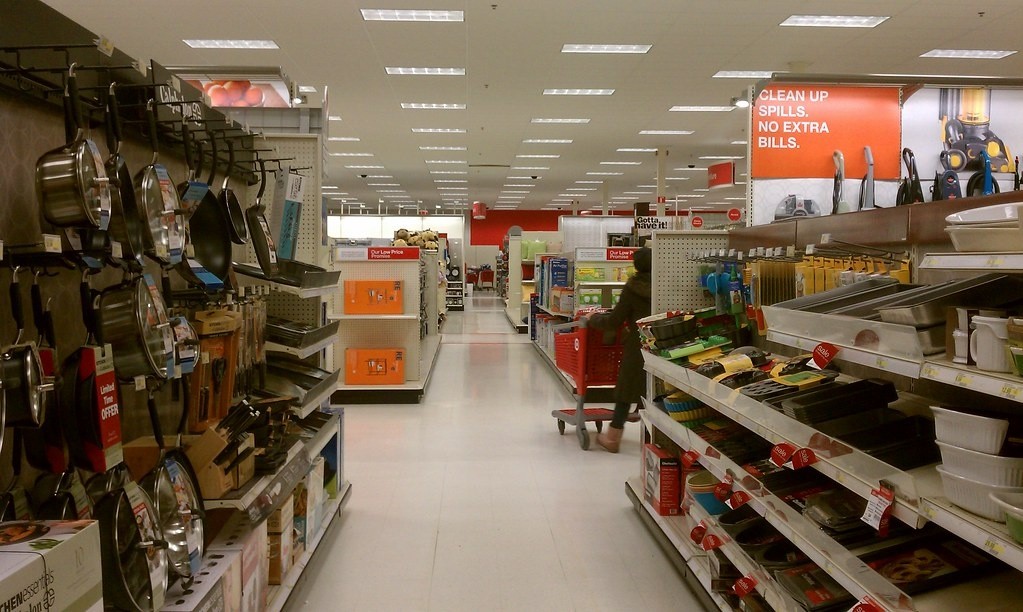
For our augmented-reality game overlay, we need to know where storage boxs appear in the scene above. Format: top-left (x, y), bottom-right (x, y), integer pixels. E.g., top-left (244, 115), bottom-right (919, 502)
top-left (0, 521), bottom-right (104, 611)
top-left (643, 443), bottom-right (680, 516)
top-left (123, 433), bottom-right (256, 489)
top-left (678, 452), bottom-right (703, 517)
top-left (533, 254), bottom-right (637, 356)
top-left (150, 454), bottom-right (323, 612)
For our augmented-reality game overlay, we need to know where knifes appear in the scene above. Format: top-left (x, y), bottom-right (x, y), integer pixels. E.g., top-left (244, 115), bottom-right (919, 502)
top-left (214, 399), bottom-right (261, 443)
top-left (233, 302), bottom-right (266, 397)
top-left (213, 431), bottom-right (255, 475)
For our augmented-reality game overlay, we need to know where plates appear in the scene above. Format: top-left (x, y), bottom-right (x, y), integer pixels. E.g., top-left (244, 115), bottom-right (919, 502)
top-left (943, 201), bottom-right (1023, 252)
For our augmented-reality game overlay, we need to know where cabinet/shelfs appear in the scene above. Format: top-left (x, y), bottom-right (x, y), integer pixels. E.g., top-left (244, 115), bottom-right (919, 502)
top-left (503, 230), bottom-right (560, 333)
top-left (155, 258), bottom-right (353, 612)
top-left (496, 252), bottom-right (509, 298)
top-left (639, 189), bottom-right (1023, 612)
top-left (532, 253), bottom-right (639, 402)
top-left (328, 231), bottom-right (464, 405)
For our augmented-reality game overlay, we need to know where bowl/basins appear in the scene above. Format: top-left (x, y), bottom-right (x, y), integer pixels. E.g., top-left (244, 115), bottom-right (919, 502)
top-left (635, 306), bottom-right (735, 349)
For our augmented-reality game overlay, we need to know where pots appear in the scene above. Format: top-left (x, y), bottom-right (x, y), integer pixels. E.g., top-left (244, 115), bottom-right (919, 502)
top-left (245, 158), bottom-right (280, 273)
top-left (101, 264), bottom-right (202, 451)
top-left (91, 376), bottom-right (207, 612)
top-left (218, 140), bottom-right (248, 244)
top-left (0, 264), bottom-right (135, 524)
top-left (32, 63), bottom-right (234, 286)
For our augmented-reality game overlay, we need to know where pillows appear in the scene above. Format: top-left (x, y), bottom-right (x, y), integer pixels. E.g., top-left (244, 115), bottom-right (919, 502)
top-left (520, 239), bottom-right (562, 261)
top-left (521, 264), bottom-right (535, 280)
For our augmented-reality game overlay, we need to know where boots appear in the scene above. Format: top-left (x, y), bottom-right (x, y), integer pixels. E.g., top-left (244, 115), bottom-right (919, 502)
top-left (597, 423), bottom-right (624, 453)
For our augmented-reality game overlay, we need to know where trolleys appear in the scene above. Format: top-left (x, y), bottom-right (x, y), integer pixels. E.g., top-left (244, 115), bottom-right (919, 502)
top-left (480, 269), bottom-right (495, 291)
top-left (551, 308), bottom-right (643, 452)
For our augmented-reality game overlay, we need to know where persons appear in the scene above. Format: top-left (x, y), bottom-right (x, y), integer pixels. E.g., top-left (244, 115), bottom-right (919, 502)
top-left (584, 246), bottom-right (651, 453)
